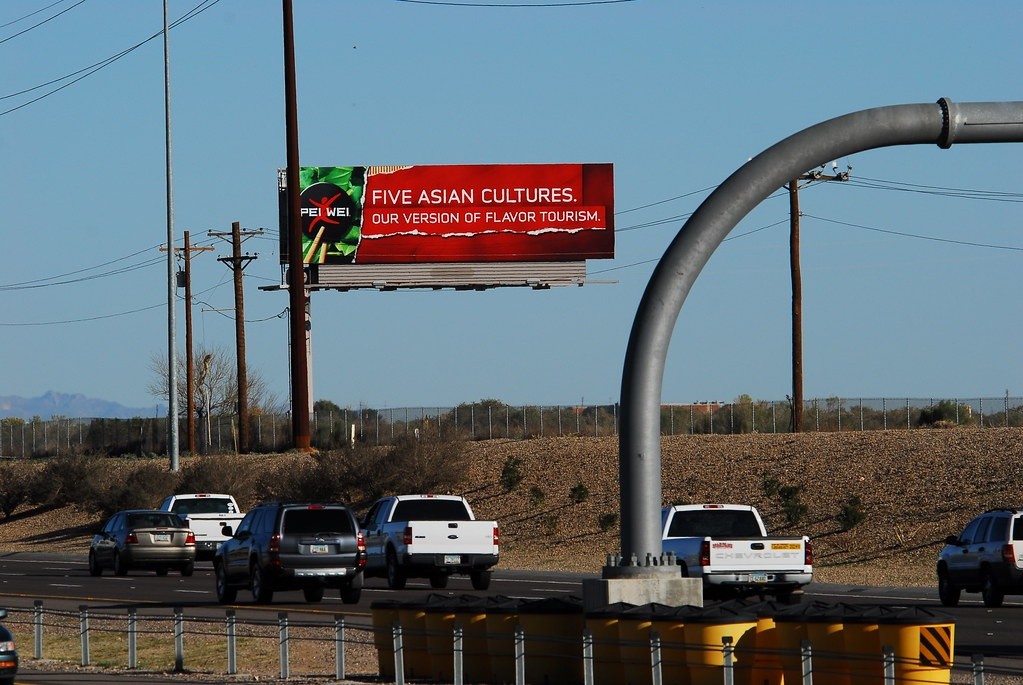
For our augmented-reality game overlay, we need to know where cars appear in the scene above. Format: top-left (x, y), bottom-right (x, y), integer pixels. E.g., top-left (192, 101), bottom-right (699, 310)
top-left (88, 509), bottom-right (197, 577)
top-left (0, 606), bottom-right (19, 685)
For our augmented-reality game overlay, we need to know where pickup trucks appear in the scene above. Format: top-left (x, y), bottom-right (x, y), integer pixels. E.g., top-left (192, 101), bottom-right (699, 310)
top-left (658, 503), bottom-right (813, 603)
top-left (159, 493), bottom-right (246, 559)
top-left (356, 493), bottom-right (500, 589)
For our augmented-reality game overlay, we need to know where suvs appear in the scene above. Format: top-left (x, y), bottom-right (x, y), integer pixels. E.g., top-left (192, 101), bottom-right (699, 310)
top-left (935, 506), bottom-right (1023, 606)
top-left (211, 499), bottom-right (368, 605)
